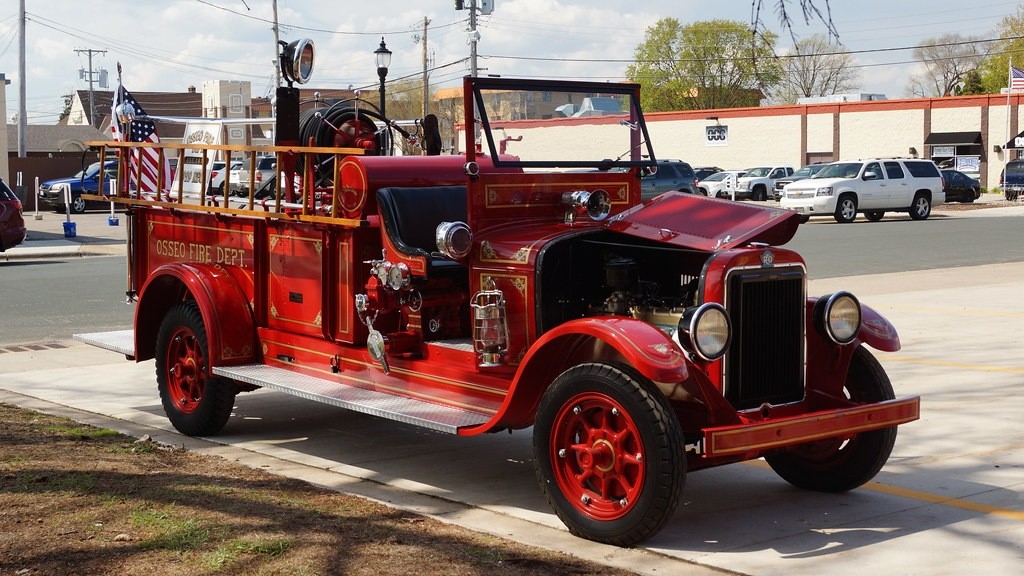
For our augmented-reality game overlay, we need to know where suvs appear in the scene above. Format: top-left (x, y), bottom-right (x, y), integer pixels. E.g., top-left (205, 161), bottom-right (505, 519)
top-left (999, 159), bottom-right (1024, 200)
top-left (780, 157), bottom-right (946, 224)
top-left (590, 159), bottom-right (700, 205)
top-left (0, 179), bottom-right (28, 252)
top-left (238, 154), bottom-right (286, 200)
top-left (38, 161), bottom-right (126, 214)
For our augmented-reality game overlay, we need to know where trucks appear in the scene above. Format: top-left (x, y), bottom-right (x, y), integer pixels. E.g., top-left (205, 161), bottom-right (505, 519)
top-left (73, 38), bottom-right (921, 546)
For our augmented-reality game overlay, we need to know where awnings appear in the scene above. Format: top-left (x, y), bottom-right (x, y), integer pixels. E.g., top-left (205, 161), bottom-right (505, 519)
top-left (924, 132), bottom-right (982, 145)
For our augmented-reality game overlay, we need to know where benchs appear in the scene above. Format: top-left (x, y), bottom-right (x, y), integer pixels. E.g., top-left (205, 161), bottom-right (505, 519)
top-left (374, 185), bottom-right (469, 277)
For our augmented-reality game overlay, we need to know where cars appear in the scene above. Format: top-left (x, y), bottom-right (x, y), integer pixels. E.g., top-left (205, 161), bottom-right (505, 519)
top-left (697, 170), bottom-right (748, 200)
top-left (939, 169), bottom-right (981, 204)
top-left (772, 161), bottom-right (834, 201)
top-left (692, 167), bottom-right (725, 181)
top-left (722, 166), bottom-right (794, 201)
top-left (212, 161), bottom-right (242, 197)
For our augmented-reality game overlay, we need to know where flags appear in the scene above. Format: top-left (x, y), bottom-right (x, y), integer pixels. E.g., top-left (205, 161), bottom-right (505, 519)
top-left (110, 84), bottom-right (171, 209)
top-left (1011, 67), bottom-right (1024, 90)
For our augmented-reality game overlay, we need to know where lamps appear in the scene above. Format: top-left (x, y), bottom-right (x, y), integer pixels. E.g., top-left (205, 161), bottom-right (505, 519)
top-left (706, 117), bottom-right (721, 126)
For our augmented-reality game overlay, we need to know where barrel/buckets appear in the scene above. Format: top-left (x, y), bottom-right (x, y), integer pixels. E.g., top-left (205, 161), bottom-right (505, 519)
top-left (63, 222), bottom-right (76, 237)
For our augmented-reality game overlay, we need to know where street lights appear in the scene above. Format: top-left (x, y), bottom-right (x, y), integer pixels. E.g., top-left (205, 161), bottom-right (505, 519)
top-left (372, 36), bottom-right (392, 123)
